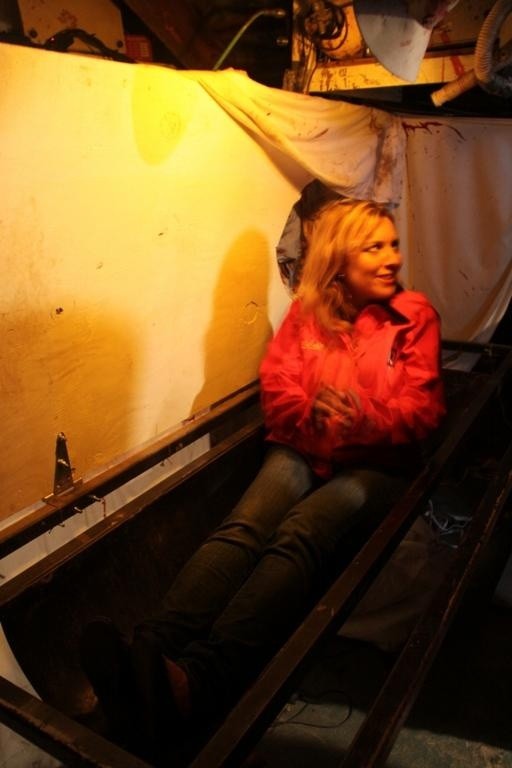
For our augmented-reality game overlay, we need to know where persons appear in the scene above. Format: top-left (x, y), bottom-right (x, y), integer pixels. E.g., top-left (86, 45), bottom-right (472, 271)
top-left (75, 198), bottom-right (447, 745)
